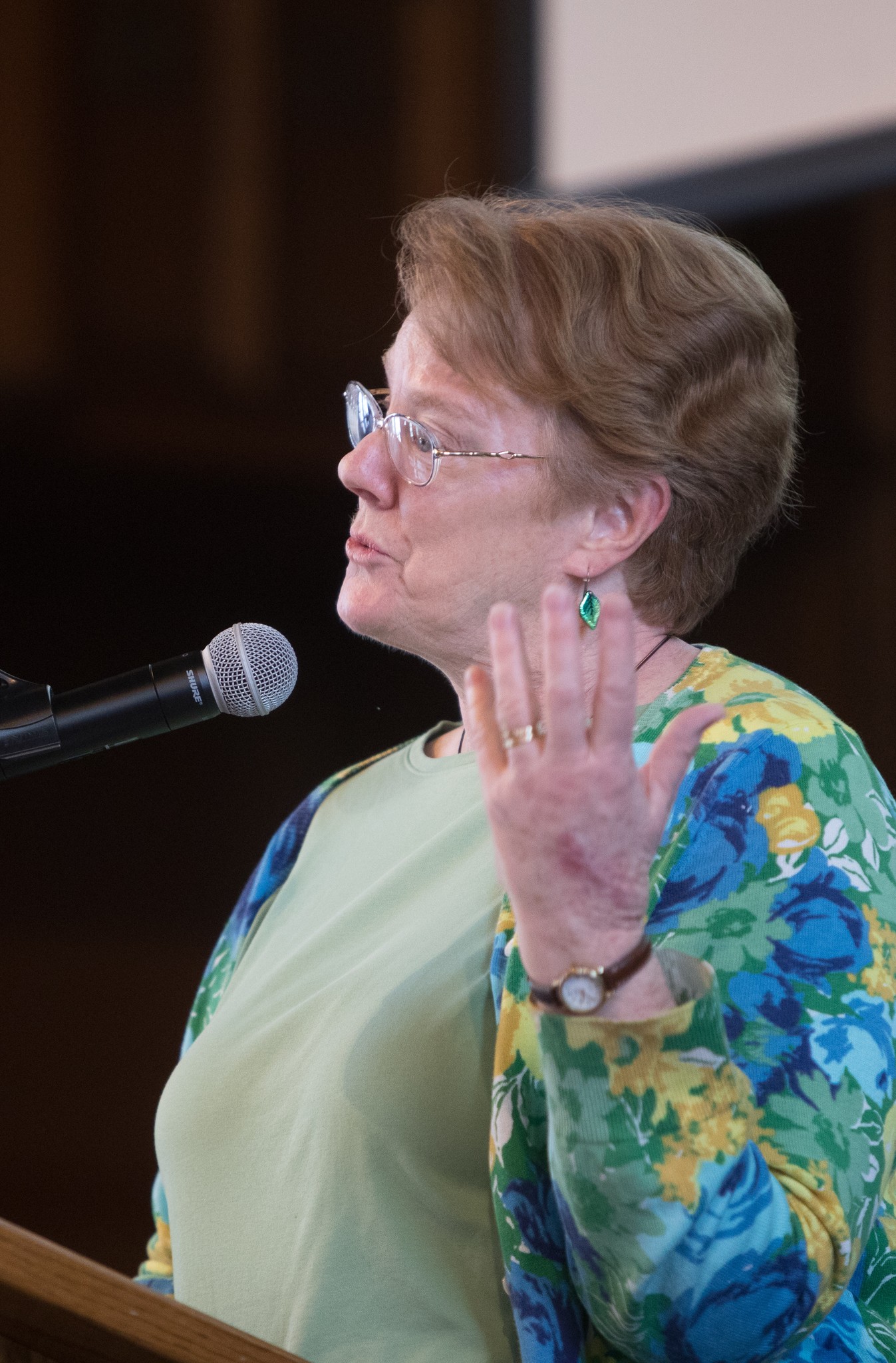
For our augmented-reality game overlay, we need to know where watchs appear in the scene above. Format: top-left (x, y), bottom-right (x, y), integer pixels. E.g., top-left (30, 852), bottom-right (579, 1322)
top-left (526, 936), bottom-right (655, 1017)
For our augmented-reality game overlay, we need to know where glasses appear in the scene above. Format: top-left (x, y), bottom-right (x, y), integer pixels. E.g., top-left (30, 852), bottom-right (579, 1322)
top-left (344, 380), bottom-right (566, 487)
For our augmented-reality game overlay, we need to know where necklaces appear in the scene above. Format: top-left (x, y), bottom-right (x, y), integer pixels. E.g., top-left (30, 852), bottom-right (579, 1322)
top-left (456, 634), bottom-right (674, 756)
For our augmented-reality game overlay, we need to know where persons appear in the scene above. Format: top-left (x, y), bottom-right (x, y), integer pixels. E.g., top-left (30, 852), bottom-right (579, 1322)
top-left (132, 185), bottom-right (896, 1363)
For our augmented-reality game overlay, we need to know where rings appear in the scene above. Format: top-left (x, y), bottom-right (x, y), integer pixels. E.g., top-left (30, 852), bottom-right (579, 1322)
top-left (499, 723), bottom-right (540, 749)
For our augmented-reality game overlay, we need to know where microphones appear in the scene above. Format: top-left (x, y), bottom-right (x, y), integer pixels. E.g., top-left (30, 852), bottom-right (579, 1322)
top-left (0, 622), bottom-right (300, 781)
